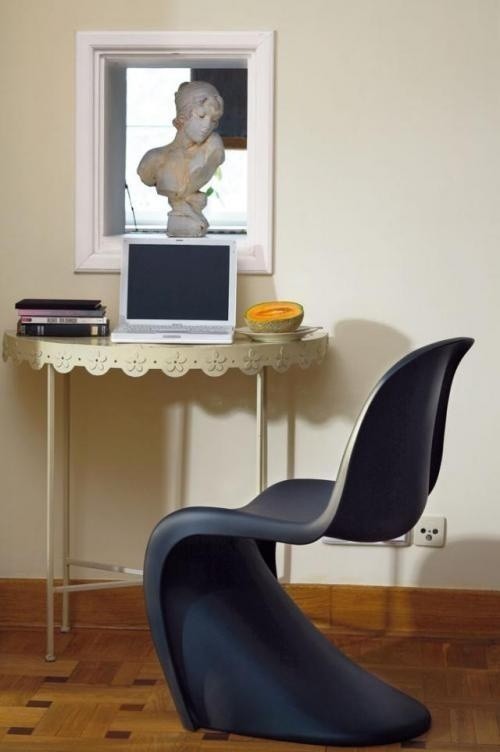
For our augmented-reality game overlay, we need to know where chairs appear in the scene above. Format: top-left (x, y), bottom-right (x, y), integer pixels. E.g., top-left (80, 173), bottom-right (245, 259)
top-left (143, 335), bottom-right (475, 746)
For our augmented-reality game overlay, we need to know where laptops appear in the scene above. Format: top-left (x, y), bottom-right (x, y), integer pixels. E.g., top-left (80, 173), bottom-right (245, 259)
top-left (110, 235), bottom-right (239, 344)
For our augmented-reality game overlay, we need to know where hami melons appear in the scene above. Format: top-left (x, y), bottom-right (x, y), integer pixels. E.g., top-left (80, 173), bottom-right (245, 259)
top-left (243, 301), bottom-right (304, 333)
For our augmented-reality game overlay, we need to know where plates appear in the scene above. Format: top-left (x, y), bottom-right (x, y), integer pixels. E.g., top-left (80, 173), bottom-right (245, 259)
top-left (236, 325), bottom-right (322, 342)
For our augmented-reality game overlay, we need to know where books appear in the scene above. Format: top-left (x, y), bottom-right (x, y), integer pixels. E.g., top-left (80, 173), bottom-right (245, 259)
top-left (15, 299), bottom-right (109, 336)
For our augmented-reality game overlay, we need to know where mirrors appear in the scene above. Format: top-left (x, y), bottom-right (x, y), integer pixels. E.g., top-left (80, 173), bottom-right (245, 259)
top-left (73, 26), bottom-right (277, 277)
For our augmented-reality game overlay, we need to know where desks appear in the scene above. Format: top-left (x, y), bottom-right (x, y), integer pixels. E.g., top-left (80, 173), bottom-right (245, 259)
top-left (4, 330), bottom-right (329, 663)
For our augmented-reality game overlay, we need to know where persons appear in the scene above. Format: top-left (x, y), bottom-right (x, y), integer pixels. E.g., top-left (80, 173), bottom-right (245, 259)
top-left (137, 81), bottom-right (225, 197)
